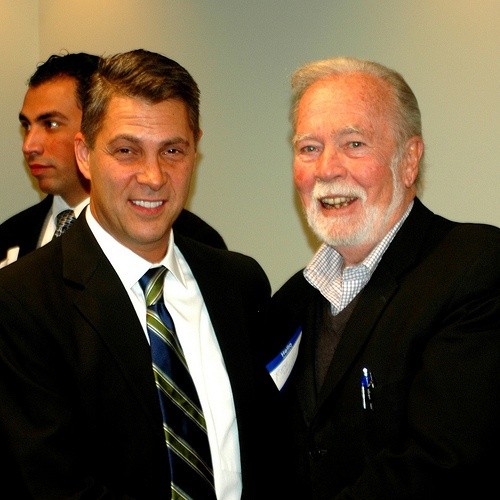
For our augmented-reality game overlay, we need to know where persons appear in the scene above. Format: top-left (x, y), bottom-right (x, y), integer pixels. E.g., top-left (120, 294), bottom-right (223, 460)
top-left (0, 53), bottom-right (105, 269)
top-left (0, 49), bottom-right (325, 500)
top-left (266, 58), bottom-right (500, 500)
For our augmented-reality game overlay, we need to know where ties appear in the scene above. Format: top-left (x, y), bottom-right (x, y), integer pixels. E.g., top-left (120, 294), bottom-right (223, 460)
top-left (52, 210), bottom-right (76, 241)
top-left (138, 265), bottom-right (217, 500)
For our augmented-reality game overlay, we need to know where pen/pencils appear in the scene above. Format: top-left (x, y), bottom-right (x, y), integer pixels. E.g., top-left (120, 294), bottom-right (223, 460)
top-left (360, 367), bottom-right (374, 412)
